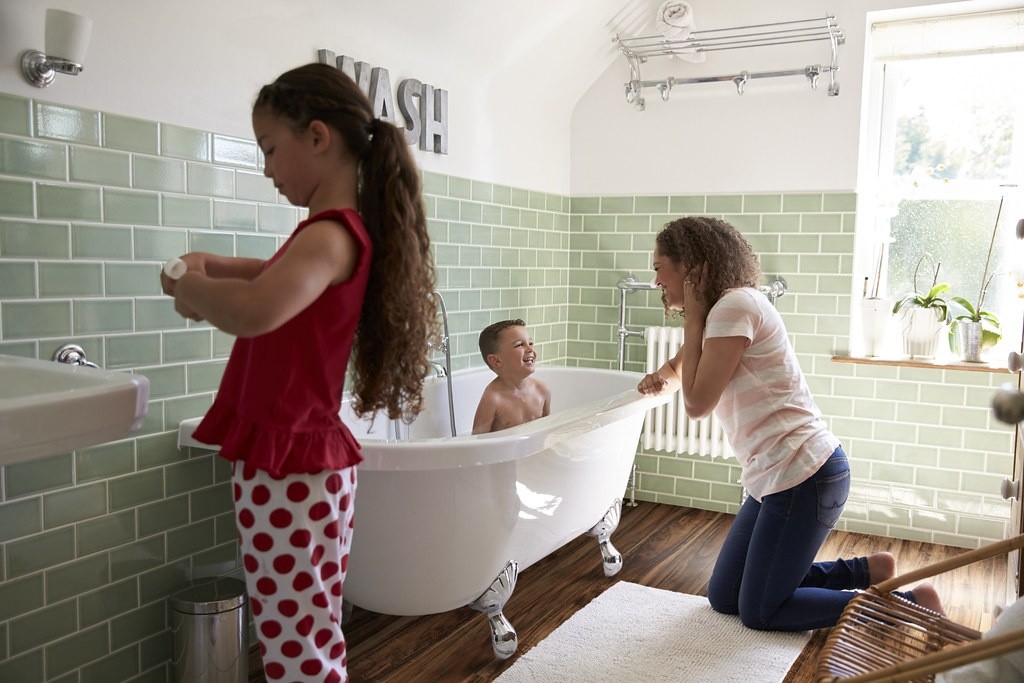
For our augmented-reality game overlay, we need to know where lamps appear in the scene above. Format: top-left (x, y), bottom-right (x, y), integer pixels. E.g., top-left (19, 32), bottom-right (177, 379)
top-left (20, 9), bottom-right (93, 88)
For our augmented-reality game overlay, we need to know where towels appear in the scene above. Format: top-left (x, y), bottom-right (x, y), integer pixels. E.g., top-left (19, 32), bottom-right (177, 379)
top-left (655, 1), bottom-right (707, 64)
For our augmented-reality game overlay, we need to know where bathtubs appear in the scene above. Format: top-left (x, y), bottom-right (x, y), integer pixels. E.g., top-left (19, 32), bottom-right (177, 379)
top-left (178, 364), bottom-right (678, 660)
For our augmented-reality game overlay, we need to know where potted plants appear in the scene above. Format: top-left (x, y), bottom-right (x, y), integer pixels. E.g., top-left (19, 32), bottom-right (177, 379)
top-left (893, 284), bottom-right (952, 362)
top-left (948, 296), bottom-right (1004, 362)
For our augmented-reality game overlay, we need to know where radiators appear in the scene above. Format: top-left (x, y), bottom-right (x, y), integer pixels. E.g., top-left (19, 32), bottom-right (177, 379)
top-left (642, 326), bottom-right (735, 459)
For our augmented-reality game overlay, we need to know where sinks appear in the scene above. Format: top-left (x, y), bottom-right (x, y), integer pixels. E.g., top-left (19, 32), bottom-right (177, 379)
top-left (1, 354), bottom-right (150, 465)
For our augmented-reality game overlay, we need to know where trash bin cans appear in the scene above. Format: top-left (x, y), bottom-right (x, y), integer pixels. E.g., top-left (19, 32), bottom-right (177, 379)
top-left (168, 578), bottom-right (251, 683)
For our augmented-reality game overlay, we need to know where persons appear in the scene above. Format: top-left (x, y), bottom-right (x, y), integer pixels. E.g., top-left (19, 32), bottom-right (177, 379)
top-left (160, 62), bottom-right (446, 682)
top-left (471, 319), bottom-right (551, 436)
top-left (638, 216), bottom-right (947, 632)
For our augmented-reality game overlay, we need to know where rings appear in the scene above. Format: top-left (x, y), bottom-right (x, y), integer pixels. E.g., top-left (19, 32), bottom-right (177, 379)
top-left (685, 281), bottom-right (691, 284)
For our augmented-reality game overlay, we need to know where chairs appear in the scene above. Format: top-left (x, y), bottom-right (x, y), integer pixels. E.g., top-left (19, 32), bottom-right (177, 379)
top-left (814, 533), bottom-right (1024, 683)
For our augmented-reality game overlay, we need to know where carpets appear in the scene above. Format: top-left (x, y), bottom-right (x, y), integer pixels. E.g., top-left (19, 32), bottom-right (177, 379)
top-left (491, 580), bottom-right (813, 683)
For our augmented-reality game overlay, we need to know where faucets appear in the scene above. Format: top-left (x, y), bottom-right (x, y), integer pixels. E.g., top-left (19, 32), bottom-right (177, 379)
top-left (430, 362), bottom-right (448, 378)
